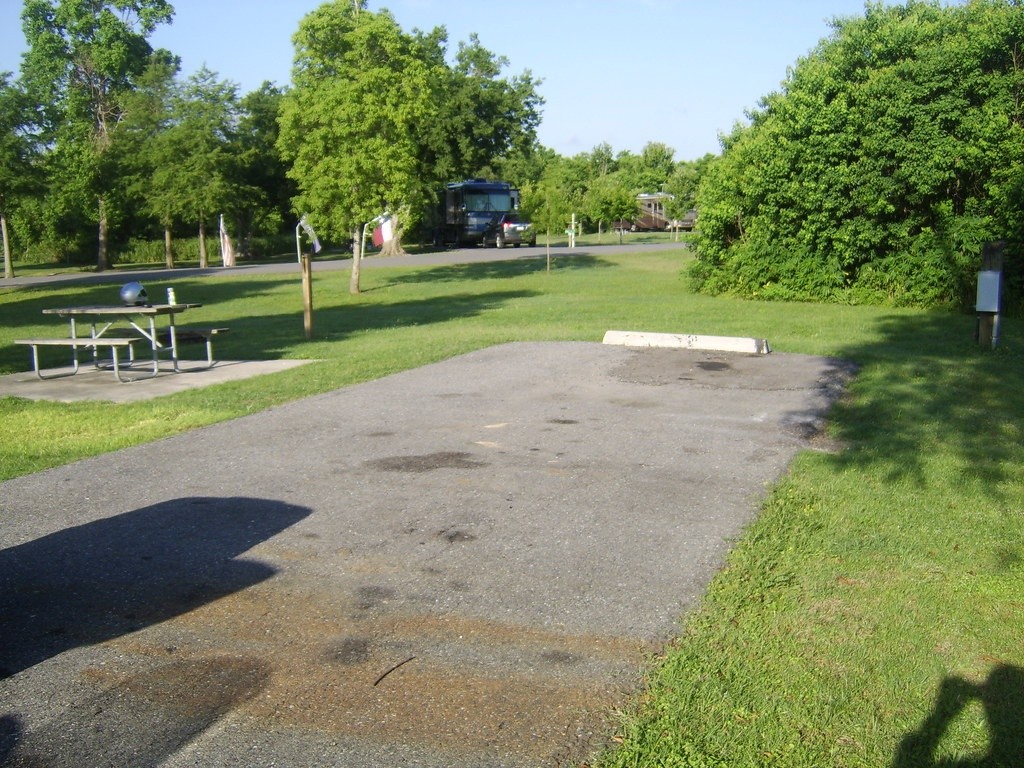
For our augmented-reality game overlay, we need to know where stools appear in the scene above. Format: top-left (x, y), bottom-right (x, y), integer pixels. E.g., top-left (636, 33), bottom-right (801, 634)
top-left (15, 338), bottom-right (142, 345)
top-left (105, 328), bottom-right (229, 336)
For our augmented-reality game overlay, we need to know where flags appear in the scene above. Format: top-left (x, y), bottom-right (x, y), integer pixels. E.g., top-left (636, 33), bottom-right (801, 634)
top-left (301, 216), bottom-right (322, 252)
top-left (372, 213), bottom-right (393, 246)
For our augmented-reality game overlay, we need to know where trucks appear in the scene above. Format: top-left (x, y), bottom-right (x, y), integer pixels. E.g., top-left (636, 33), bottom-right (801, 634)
top-left (427, 178), bottom-right (521, 250)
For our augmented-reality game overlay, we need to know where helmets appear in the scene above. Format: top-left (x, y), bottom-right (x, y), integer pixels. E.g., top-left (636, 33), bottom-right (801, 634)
top-left (120, 281), bottom-right (150, 306)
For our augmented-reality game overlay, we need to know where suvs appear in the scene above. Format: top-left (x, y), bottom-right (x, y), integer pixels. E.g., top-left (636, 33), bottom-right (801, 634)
top-left (481, 211), bottom-right (537, 249)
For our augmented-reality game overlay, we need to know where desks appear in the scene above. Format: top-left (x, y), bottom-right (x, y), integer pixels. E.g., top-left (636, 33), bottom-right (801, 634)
top-left (14, 304), bottom-right (229, 382)
